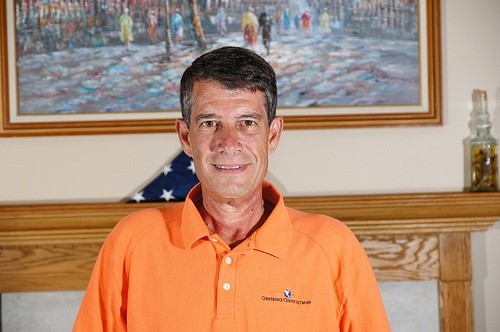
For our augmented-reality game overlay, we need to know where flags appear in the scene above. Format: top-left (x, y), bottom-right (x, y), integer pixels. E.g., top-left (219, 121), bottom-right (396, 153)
top-left (125, 150), bottom-right (199, 204)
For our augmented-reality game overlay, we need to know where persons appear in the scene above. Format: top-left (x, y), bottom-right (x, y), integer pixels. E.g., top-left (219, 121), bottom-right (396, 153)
top-left (71, 46), bottom-right (393, 332)
top-left (21, 5), bottom-right (331, 56)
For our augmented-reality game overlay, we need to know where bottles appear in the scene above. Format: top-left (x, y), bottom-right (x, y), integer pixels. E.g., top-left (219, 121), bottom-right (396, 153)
top-left (470, 90), bottom-right (496, 191)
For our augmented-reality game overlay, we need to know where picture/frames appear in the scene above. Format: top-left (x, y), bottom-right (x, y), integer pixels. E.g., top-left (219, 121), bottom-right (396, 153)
top-left (0, 0), bottom-right (443, 138)
